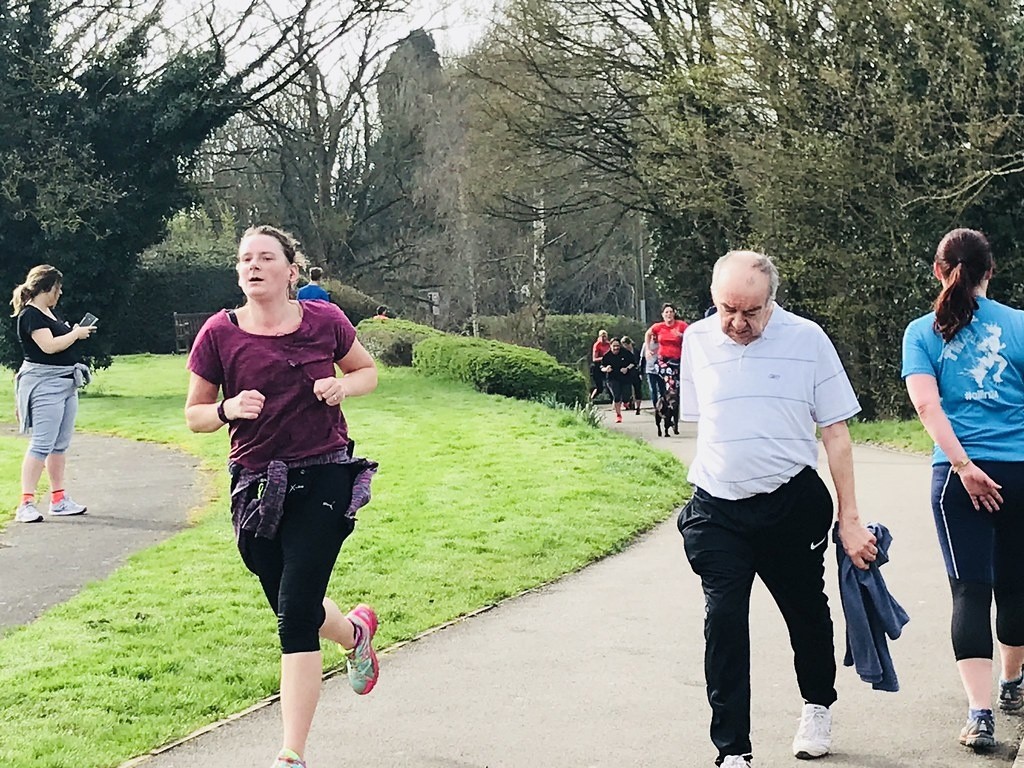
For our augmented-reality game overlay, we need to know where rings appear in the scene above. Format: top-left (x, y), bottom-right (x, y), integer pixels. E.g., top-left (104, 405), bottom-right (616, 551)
top-left (333, 394), bottom-right (338, 401)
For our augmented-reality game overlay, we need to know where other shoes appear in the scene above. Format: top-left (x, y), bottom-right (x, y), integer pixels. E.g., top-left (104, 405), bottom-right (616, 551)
top-left (635, 407), bottom-right (640, 415)
top-left (616, 415), bottom-right (622, 423)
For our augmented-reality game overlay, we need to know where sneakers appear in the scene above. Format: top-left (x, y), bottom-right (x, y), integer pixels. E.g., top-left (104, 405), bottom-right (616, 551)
top-left (960, 708), bottom-right (995, 747)
top-left (273, 748), bottom-right (307, 768)
top-left (721, 753), bottom-right (752, 768)
top-left (48, 496), bottom-right (88, 515)
top-left (339, 604), bottom-right (379, 695)
top-left (793, 701), bottom-right (832, 760)
top-left (14, 505), bottom-right (43, 523)
top-left (998, 665), bottom-right (1024, 710)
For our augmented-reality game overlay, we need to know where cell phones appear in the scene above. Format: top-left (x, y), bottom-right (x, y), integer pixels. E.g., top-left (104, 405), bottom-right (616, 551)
top-left (80, 312), bottom-right (99, 327)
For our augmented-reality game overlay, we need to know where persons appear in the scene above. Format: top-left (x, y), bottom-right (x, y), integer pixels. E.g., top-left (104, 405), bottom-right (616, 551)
top-left (705, 281), bottom-right (718, 317)
top-left (620, 336), bottom-right (641, 415)
top-left (676, 251), bottom-right (879, 768)
top-left (600, 337), bottom-right (636, 423)
top-left (374, 305), bottom-right (387, 319)
top-left (10, 264), bottom-right (97, 523)
top-left (902, 226), bottom-right (1024, 747)
top-left (589, 330), bottom-right (610, 408)
top-left (636, 303), bottom-right (690, 437)
top-left (297, 267), bottom-right (330, 300)
top-left (184, 225), bottom-right (379, 768)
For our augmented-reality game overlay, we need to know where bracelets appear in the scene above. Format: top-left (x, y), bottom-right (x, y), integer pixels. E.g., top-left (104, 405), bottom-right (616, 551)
top-left (950, 457), bottom-right (971, 474)
top-left (217, 396), bottom-right (228, 424)
top-left (626, 366), bottom-right (631, 372)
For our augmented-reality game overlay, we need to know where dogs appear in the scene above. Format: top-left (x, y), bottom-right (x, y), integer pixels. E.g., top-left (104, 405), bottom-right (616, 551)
top-left (655, 386), bottom-right (680, 437)
